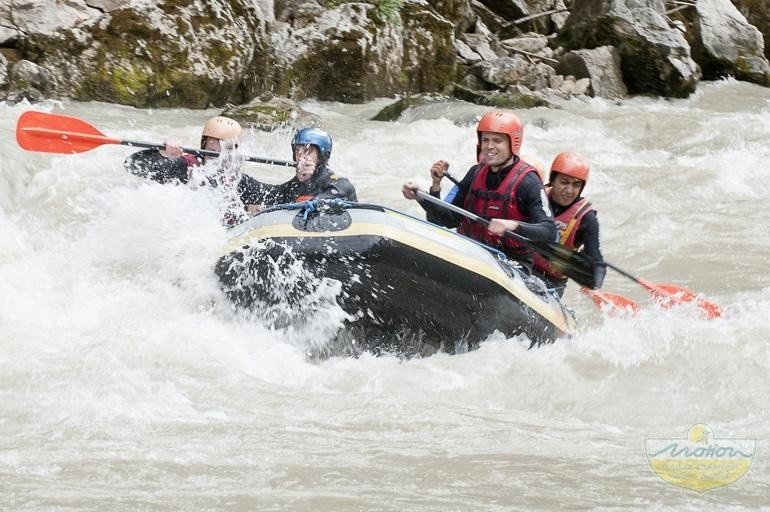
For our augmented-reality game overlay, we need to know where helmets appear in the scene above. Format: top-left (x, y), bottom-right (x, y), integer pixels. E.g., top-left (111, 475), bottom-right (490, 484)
top-left (549, 150), bottom-right (590, 196)
top-left (200, 115), bottom-right (243, 150)
top-left (291, 126), bottom-right (333, 162)
top-left (476, 110), bottom-right (522, 162)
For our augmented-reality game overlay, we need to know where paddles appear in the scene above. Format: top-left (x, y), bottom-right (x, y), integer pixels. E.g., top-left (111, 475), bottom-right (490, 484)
top-left (16, 112), bottom-right (300, 169)
top-left (406, 181), bottom-right (596, 290)
top-left (438, 162), bottom-right (722, 323)
top-left (572, 278), bottom-right (640, 322)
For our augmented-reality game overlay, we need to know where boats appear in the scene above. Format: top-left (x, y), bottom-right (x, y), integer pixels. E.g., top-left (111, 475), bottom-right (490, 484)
top-left (210, 197), bottom-right (590, 363)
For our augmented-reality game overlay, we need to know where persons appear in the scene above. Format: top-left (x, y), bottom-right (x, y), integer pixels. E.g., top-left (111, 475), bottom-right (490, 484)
top-left (124, 115), bottom-right (269, 218)
top-left (218, 127), bottom-right (358, 229)
top-left (421, 139), bottom-right (487, 229)
top-left (522, 151), bottom-right (607, 298)
top-left (402, 110), bottom-right (557, 278)
